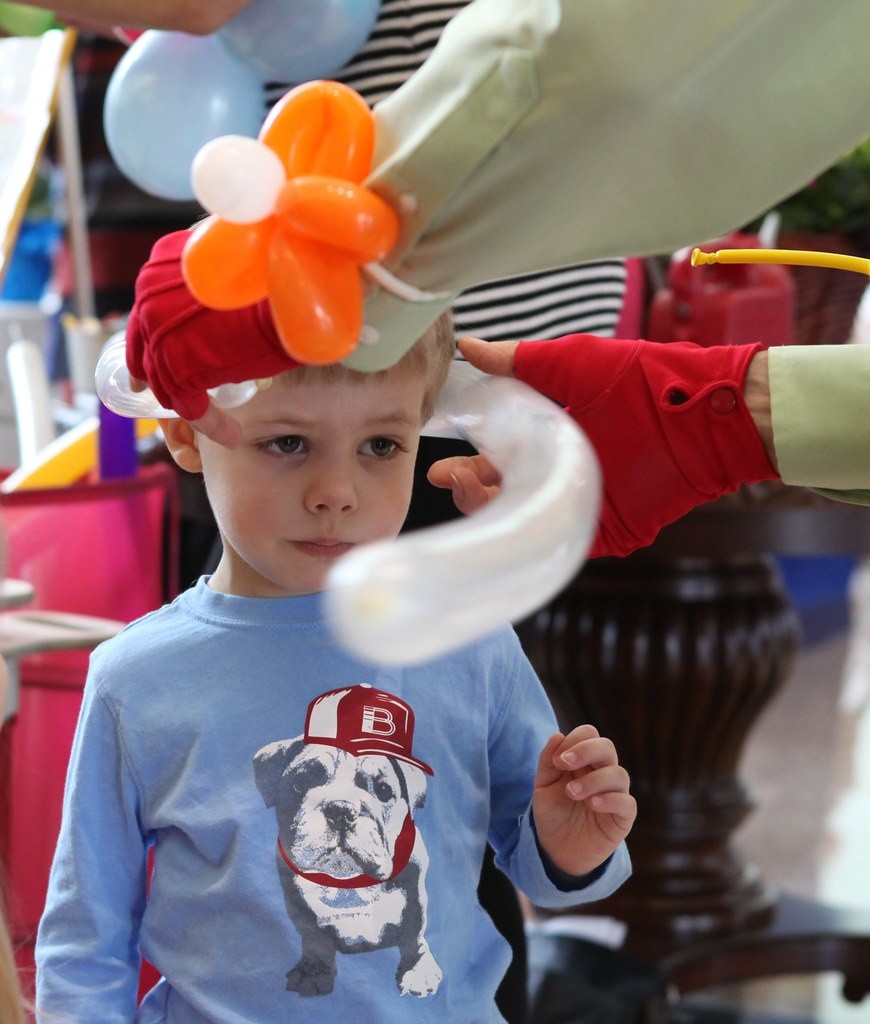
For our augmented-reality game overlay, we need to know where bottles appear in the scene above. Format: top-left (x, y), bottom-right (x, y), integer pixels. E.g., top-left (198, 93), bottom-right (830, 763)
top-left (650, 236), bottom-right (793, 347)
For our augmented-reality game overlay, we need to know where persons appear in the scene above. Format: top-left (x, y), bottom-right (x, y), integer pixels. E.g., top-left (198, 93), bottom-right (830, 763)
top-left (12, 0), bottom-right (627, 356)
top-left (132, 0), bottom-right (870, 563)
top-left (33, 312), bottom-right (637, 1024)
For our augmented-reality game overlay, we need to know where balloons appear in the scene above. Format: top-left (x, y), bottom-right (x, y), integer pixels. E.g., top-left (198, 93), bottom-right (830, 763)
top-left (0, 409), bottom-right (159, 495)
top-left (178, 79), bottom-right (396, 368)
top-left (105, 0), bottom-right (382, 200)
top-left (91, 332), bottom-right (601, 665)
